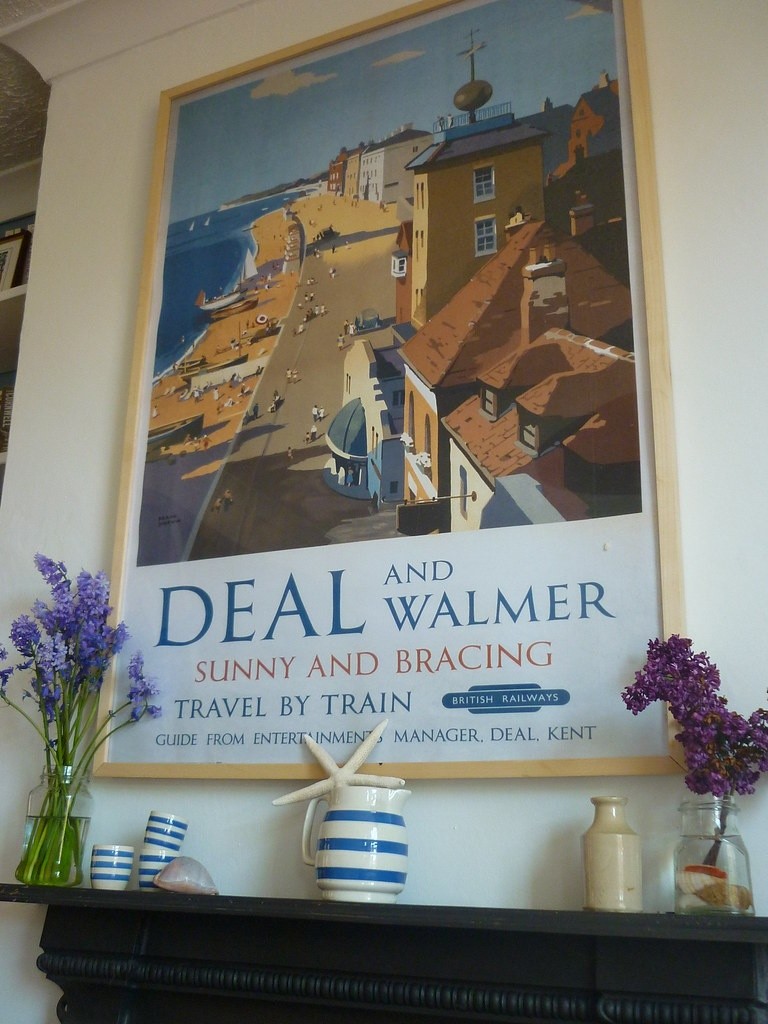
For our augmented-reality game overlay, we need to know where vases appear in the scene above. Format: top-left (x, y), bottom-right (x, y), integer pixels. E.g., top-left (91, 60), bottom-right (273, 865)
top-left (13, 767), bottom-right (92, 889)
top-left (671, 797), bottom-right (754, 917)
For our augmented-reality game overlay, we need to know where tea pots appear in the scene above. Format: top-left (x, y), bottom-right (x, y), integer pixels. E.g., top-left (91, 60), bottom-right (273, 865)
top-left (300, 783), bottom-right (412, 905)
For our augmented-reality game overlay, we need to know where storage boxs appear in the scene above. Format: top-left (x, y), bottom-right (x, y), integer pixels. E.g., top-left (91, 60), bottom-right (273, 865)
top-left (582, 795), bottom-right (648, 912)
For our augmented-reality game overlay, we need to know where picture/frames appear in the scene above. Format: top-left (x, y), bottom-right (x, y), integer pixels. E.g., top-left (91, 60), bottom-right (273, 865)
top-left (89, 1), bottom-right (698, 780)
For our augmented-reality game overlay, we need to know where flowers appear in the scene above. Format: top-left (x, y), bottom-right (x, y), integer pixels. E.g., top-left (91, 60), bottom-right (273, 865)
top-left (1, 550), bottom-right (165, 766)
top-left (623, 634), bottom-right (768, 797)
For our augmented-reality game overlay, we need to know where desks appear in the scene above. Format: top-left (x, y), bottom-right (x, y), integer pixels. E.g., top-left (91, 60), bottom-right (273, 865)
top-left (1, 881), bottom-right (767, 1024)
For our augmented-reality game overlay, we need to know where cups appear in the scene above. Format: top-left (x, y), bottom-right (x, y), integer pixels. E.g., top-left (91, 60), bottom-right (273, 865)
top-left (137, 847), bottom-right (182, 893)
top-left (144, 807), bottom-right (189, 856)
top-left (89, 843), bottom-right (137, 893)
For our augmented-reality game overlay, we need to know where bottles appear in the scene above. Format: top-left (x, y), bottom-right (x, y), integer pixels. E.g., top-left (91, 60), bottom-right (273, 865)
top-left (581, 794), bottom-right (645, 915)
